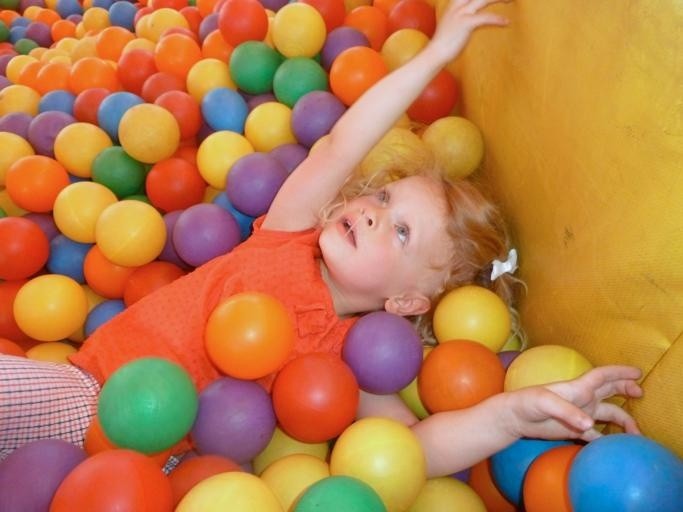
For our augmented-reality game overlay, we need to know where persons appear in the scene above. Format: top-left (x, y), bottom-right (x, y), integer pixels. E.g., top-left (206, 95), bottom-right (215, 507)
top-left (0, 1), bottom-right (645, 479)
